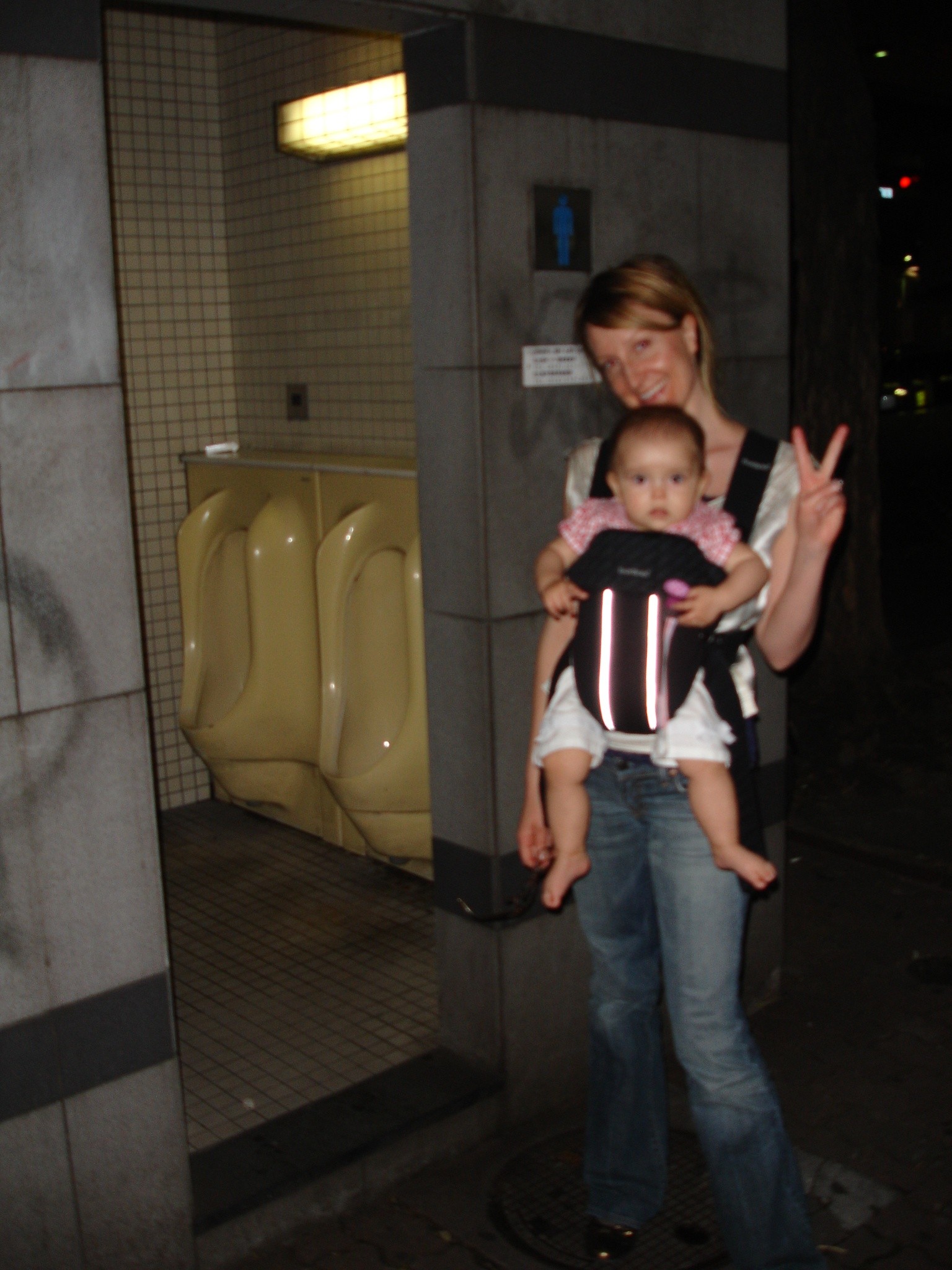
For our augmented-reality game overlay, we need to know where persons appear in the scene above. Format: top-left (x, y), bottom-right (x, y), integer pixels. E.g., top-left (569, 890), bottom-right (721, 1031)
top-left (532, 401), bottom-right (778, 909)
top-left (518, 253), bottom-right (850, 1270)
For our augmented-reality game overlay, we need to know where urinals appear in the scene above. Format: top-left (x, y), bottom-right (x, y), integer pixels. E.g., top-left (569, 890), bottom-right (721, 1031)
top-left (173, 445), bottom-right (318, 841)
top-left (312, 451), bottom-right (434, 882)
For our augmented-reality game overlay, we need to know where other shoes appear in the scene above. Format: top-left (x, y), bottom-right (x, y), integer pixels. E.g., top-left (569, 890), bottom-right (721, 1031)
top-left (585, 1214), bottom-right (639, 1258)
